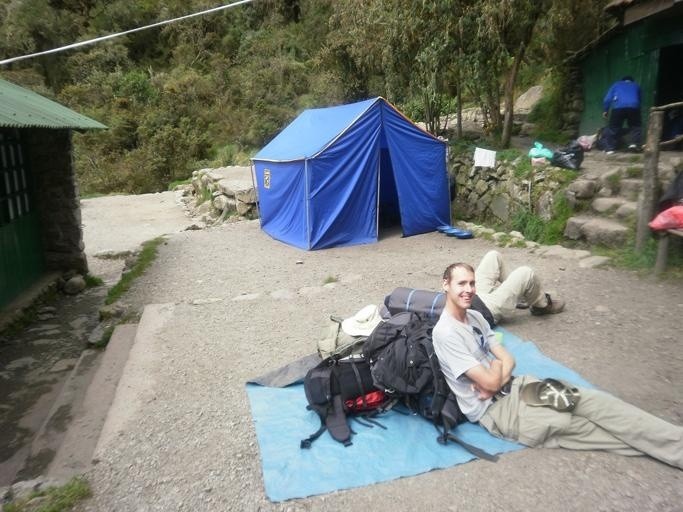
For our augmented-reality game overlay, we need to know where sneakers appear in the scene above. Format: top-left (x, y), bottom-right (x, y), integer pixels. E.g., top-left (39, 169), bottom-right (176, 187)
top-left (517, 293), bottom-right (566, 316)
top-left (628, 144), bottom-right (644, 152)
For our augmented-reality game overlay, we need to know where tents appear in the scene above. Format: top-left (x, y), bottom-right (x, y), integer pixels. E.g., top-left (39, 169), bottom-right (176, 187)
top-left (247, 94), bottom-right (453, 251)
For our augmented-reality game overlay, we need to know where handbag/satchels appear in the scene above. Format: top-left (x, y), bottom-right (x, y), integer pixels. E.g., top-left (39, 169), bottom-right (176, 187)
top-left (300, 354), bottom-right (389, 449)
top-left (379, 286), bottom-right (448, 323)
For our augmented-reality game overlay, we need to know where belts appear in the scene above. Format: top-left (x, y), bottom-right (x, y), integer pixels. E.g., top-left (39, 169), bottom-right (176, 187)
top-left (492, 375), bottom-right (514, 401)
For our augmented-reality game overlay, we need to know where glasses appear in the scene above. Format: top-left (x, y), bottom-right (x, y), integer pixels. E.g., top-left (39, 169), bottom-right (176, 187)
top-left (471, 325), bottom-right (483, 348)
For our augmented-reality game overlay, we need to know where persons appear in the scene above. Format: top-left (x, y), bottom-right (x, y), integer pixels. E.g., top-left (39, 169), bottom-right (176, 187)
top-left (469, 249), bottom-right (567, 329)
top-left (430, 263), bottom-right (683, 471)
top-left (601, 75), bottom-right (643, 155)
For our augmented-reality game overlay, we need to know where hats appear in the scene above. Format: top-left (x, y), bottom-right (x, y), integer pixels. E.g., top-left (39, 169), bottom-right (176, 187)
top-left (340, 304), bottom-right (385, 336)
top-left (520, 377), bottom-right (582, 413)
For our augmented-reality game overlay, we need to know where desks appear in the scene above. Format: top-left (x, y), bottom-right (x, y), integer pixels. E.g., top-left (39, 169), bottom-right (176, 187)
top-left (655, 228), bottom-right (683, 274)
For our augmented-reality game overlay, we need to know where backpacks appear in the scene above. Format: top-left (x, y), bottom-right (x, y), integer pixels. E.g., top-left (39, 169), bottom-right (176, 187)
top-left (362, 312), bottom-right (500, 463)
